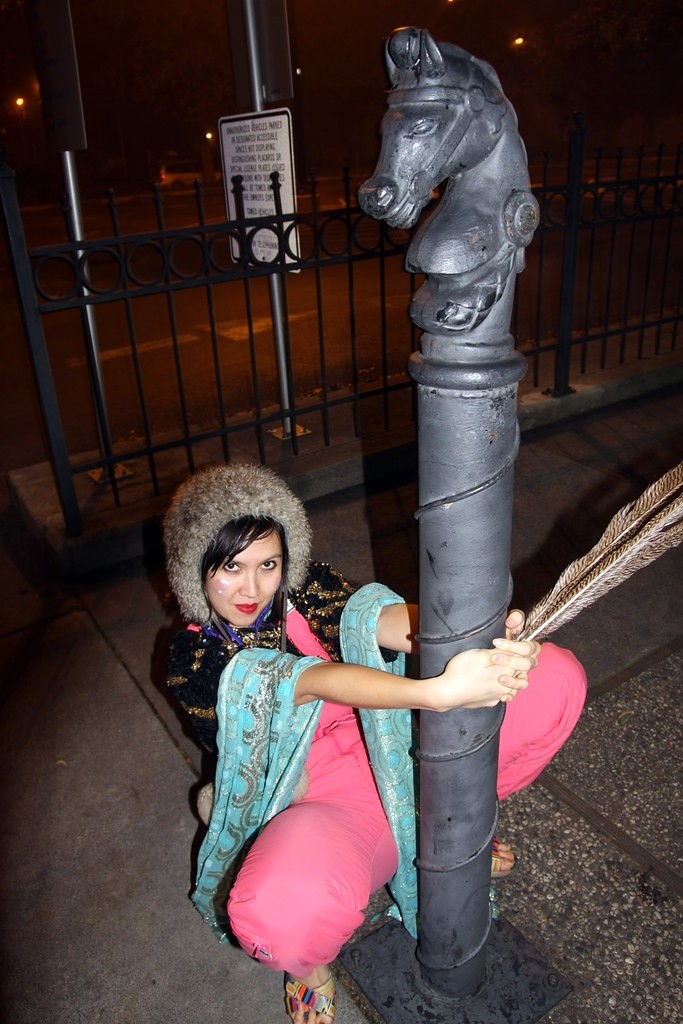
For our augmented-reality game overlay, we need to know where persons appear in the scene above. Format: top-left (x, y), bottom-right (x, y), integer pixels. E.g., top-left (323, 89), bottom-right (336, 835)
top-left (161, 462), bottom-right (587, 1024)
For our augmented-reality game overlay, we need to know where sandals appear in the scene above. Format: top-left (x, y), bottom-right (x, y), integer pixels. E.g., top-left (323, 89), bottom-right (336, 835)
top-left (284, 974), bottom-right (337, 1024)
top-left (490, 836), bottom-right (514, 877)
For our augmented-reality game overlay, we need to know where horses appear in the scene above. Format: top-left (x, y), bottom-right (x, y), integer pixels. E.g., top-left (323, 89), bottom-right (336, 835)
top-left (353, 35), bottom-right (545, 285)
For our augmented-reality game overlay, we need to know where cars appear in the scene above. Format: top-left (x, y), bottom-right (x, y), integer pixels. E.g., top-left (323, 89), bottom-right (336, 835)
top-left (150, 159), bottom-right (222, 188)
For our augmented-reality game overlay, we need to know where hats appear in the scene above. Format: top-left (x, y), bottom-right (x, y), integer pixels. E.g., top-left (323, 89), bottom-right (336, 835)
top-left (159, 463), bottom-right (310, 627)
